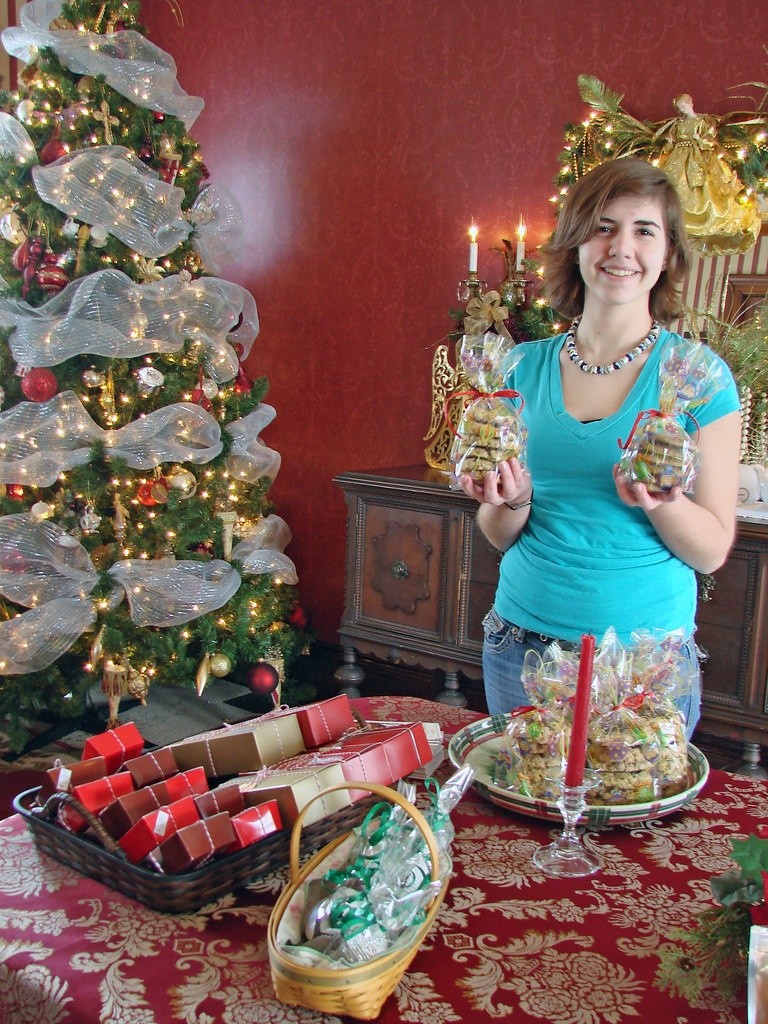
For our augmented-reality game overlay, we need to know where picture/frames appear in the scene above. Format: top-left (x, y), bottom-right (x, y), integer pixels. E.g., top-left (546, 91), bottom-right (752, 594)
top-left (724, 274), bottom-right (768, 329)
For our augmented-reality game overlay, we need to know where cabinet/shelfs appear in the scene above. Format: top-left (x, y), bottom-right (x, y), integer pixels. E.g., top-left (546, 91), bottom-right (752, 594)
top-left (331, 463), bottom-right (768, 785)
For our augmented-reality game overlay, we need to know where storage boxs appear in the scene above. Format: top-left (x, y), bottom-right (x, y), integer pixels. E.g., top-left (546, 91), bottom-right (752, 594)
top-left (28, 694), bottom-right (433, 877)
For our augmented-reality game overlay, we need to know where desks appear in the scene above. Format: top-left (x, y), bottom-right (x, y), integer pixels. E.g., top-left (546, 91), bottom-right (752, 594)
top-left (0, 695), bottom-right (768, 1024)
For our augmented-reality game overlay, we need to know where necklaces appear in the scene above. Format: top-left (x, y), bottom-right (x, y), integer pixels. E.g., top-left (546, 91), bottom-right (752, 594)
top-left (567, 311), bottom-right (660, 375)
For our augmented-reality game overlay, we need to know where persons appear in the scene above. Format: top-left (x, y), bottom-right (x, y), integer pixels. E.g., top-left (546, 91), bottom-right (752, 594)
top-left (459, 157), bottom-right (742, 741)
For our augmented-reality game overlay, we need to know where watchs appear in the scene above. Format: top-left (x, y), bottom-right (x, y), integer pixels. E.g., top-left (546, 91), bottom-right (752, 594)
top-left (504, 487), bottom-right (534, 511)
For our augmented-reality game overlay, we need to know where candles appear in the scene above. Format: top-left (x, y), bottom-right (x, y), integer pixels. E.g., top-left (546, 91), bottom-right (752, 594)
top-left (516, 241), bottom-right (525, 270)
top-left (564, 633), bottom-right (594, 787)
top-left (470, 242), bottom-right (478, 272)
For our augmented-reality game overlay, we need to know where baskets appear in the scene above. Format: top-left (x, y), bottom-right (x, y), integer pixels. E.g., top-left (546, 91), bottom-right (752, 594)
top-left (14, 708), bottom-right (410, 914)
top-left (266, 782), bottom-right (451, 1021)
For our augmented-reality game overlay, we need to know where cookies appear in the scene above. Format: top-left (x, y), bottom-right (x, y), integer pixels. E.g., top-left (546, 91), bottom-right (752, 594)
top-left (452, 406), bottom-right (523, 487)
top-left (617, 425), bottom-right (696, 494)
top-left (505, 695), bottom-right (692, 807)
